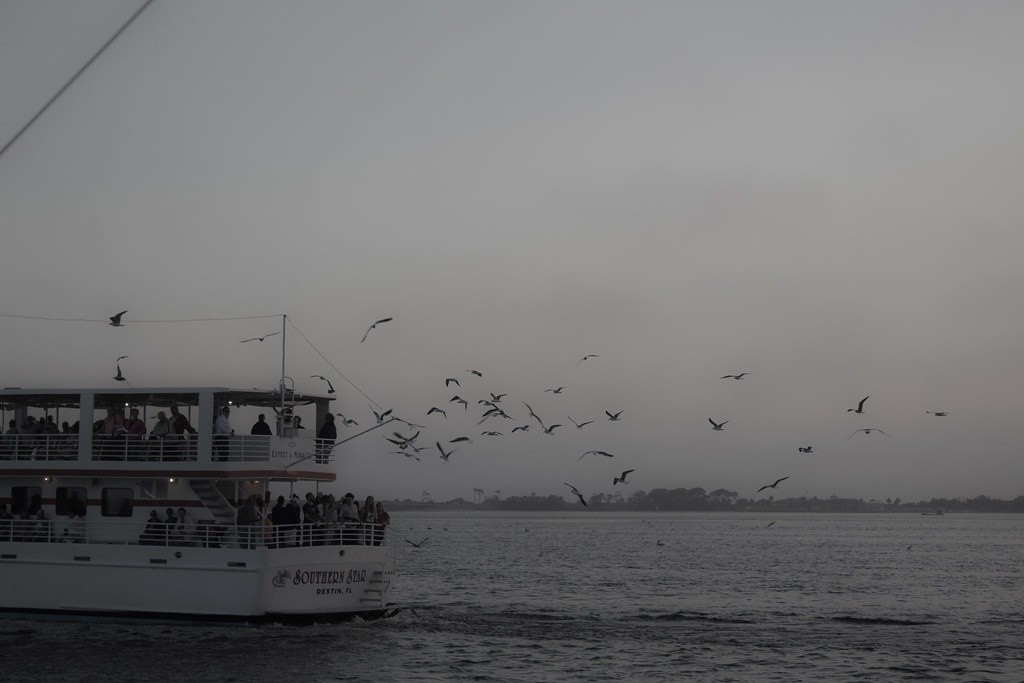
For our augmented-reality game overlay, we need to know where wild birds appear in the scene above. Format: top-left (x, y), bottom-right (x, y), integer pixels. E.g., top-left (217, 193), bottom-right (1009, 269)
top-left (109, 312), bottom-right (948, 572)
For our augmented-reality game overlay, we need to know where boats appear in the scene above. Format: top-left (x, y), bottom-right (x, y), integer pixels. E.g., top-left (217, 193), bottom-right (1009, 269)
top-left (1, 312), bottom-right (411, 625)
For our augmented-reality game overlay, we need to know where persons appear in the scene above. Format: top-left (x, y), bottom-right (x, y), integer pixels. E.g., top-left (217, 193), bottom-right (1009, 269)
top-left (251, 413), bottom-right (273, 436)
top-left (275, 409), bottom-right (304, 437)
top-left (315, 412), bottom-right (337, 462)
top-left (0, 497), bottom-right (87, 542)
top-left (151, 400), bottom-right (198, 461)
top-left (223, 491), bottom-right (390, 546)
top-left (139, 507), bottom-right (197, 545)
top-left (0, 405), bottom-right (146, 460)
top-left (212, 406), bottom-right (235, 460)
